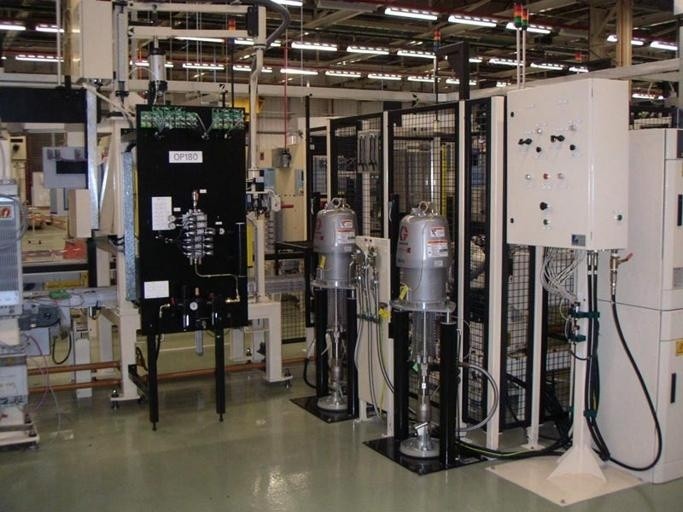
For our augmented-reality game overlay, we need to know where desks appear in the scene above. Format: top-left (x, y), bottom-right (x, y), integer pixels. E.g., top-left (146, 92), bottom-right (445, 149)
top-left (94, 295), bottom-right (293, 410)
top-left (0, 326), bottom-right (50, 452)
top-left (93, 236), bottom-right (134, 314)
top-left (26, 214), bottom-right (41, 244)
